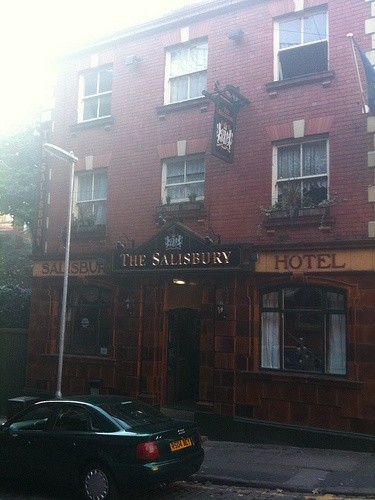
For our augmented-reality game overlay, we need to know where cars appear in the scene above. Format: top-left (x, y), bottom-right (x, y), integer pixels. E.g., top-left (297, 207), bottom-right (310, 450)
top-left (0, 394), bottom-right (204, 500)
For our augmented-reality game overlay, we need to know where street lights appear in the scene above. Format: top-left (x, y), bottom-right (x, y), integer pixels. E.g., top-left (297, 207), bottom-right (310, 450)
top-left (42, 144), bottom-right (79, 399)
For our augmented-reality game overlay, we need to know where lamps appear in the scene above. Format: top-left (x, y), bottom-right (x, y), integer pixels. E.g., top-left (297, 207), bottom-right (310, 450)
top-left (158, 207), bottom-right (171, 224)
top-left (116, 232), bottom-right (136, 250)
top-left (125, 55), bottom-right (140, 66)
top-left (229, 28), bottom-right (242, 41)
top-left (205, 226), bottom-right (221, 244)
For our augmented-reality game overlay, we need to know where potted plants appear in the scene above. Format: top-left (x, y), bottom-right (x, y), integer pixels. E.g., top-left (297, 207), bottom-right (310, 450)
top-left (259, 200), bottom-right (292, 219)
top-left (294, 198), bottom-right (330, 217)
